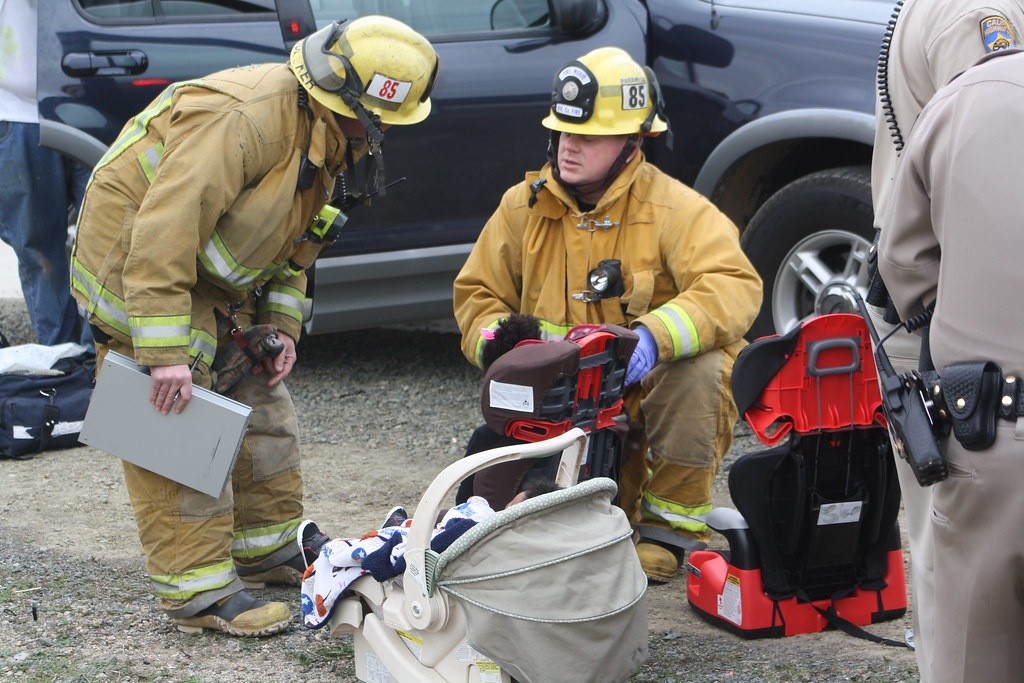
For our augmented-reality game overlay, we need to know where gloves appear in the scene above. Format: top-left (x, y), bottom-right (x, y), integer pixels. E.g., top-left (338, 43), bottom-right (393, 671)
top-left (623, 327), bottom-right (659, 387)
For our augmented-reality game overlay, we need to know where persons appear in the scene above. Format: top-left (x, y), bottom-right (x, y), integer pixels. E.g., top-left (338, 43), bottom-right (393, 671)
top-left (866, 0), bottom-right (1024, 683)
top-left (0, 1), bottom-right (95, 368)
top-left (455, 46), bottom-right (765, 584)
top-left (68, 16), bottom-right (439, 637)
top-left (877, 42), bottom-right (1024, 683)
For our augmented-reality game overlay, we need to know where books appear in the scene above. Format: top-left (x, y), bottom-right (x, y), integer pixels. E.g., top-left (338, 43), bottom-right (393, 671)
top-left (79, 351), bottom-right (255, 500)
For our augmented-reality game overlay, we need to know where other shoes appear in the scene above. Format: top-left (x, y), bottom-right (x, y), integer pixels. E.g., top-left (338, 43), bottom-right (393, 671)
top-left (175, 590), bottom-right (293, 636)
top-left (297, 520), bottom-right (332, 568)
top-left (381, 506), bottom-right (408, 529)
top-left (634, 537), bottom-right (685, 582)
top-left (239, 552), bottom-right (306, 588)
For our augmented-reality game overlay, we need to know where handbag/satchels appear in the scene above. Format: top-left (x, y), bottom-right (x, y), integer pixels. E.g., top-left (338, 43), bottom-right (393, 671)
top-left (1, 357), bottom-right (92, 460)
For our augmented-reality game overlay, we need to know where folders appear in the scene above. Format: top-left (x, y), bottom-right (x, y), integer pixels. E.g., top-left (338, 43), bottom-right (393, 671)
top-left (77, 348), bottom-right (253, 500)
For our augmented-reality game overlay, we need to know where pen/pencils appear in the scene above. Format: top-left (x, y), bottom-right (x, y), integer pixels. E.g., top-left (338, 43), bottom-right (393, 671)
top-left (172, 351), bottom-right (204, 402)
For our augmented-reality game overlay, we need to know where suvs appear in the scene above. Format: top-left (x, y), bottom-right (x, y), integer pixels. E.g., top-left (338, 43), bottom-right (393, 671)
top-left (37, 2), bottom-right (892, 338)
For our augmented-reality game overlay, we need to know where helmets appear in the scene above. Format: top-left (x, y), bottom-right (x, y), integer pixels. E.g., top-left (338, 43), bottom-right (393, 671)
top-left (290, 15), bottom-right (440, 125)
top-left (542, 47), bottom-right (668, 135)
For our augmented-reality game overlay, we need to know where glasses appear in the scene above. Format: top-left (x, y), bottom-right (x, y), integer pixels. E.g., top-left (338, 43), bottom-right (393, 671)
top-left (302, 18), bottom-right (384, 143)
